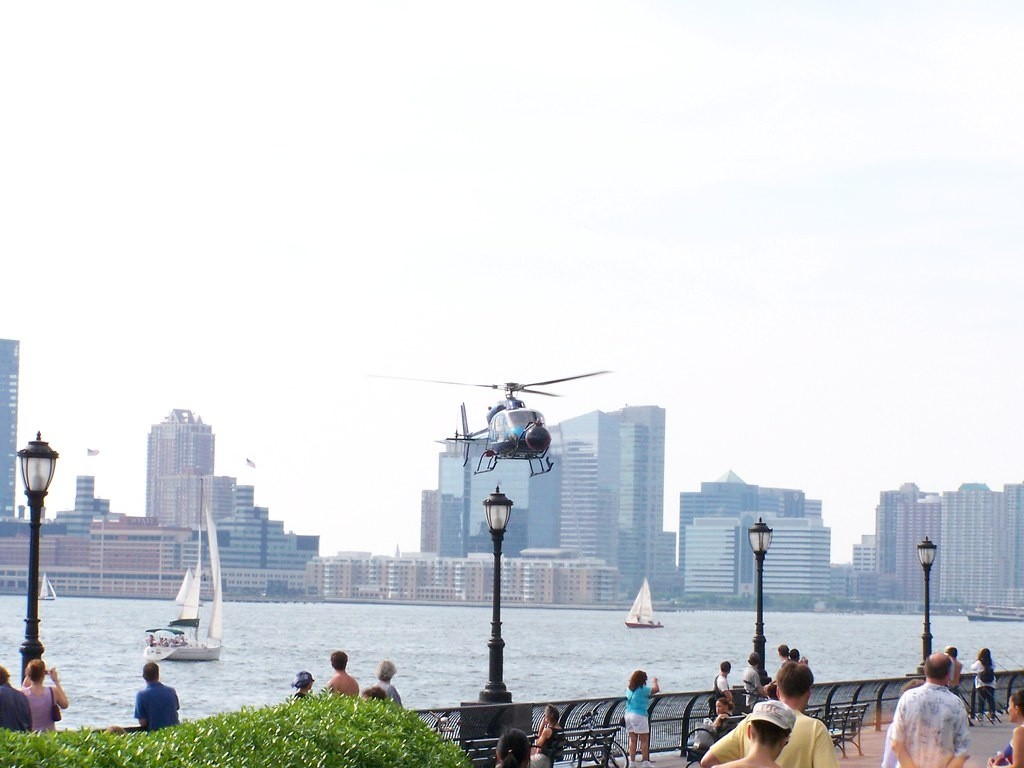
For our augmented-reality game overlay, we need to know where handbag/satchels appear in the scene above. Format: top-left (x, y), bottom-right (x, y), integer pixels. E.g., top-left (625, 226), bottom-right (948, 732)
top-left (714, 689), bottom-right (727, 699)
top-left (49, 687), bottom-right (62, 722)
top-left (693, 722), bottom-right (716, 750)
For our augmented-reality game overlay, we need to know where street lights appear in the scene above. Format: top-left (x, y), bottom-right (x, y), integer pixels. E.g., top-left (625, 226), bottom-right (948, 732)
top-left (15, 430), bottom-right (59, 684)
top-left (917, 536), bottom-right (938, 675)
top-left (479, 485), bottom-right (514, 701)
top-left (748, 517), bottom-right (773, 686)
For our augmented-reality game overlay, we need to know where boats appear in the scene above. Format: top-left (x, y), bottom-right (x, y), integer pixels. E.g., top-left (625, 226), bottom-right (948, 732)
top-left (966, 604), bottom-right (1024, 622)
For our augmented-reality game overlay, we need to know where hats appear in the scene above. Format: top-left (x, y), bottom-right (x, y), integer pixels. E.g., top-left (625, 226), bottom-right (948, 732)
top-left (747, 700), bottom-right (797, 733)
top-left (291, 671), bottom-right (315, 688)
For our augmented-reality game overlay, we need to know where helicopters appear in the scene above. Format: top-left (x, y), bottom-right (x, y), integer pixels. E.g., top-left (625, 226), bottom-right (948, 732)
top-left (365, 371), bottom-right (610, 478)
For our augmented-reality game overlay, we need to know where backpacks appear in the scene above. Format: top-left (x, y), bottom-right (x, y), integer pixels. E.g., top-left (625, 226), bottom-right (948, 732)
top-left (978, 658), bottom-right (995, 683)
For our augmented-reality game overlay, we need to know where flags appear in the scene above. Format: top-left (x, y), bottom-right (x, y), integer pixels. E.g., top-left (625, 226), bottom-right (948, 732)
top-left (87, 449), bottom-right (99, 456)
top-left (246, 459), bottom-right (255, 467)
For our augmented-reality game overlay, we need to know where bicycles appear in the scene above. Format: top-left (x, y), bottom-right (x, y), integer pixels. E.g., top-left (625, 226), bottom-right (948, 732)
top-left (576, 701), bottom-right (630, 768)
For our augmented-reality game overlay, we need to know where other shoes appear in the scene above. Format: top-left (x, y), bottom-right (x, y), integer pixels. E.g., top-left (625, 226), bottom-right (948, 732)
top-left (987, 713), bottom-right (995, 721)
top-left (976, 715), bottom-right (985, 721)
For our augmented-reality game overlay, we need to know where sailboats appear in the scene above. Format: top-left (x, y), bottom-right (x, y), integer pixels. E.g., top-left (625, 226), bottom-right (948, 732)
top-left (624, 577), bottom-right (664, 629)
top-left (144, 478), bottom-right (224, 662)
top-left (38, 572), bottom-right (56, 600)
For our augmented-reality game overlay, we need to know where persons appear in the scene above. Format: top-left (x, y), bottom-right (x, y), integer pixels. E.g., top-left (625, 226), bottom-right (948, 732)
top-left (372, 659), bottom-right (402, 707)
top-left (988, 689), bottom-right (1024, 767)
top-left (711, 700), bottom-right (796, 768)
top-left (970, 647), bottom-right (997, 722)
top-left (882, 679), bottom-right (926, 768)
top-left (361, 687), bottom-right (386, 700)
top-left (134, 663), bottom-right (180, 731)
top-left (944, 646), bottom-right (962, 697)
top-left (17, 659), bottom-right (69, 733)
top-left (326, 651), bottom-right (359, 699)
top-left (700, 661), bottom-right (839, 768)
top-left (494, 728), bottom-right (534, 768)
top-left (147, 632), bottom-right (185, 647)
top-left (889, 653), bottom-right (971, 768)
top-left (291, 671), bottom-right (314, 698)
top-left (529, 704), bottom-right (565, 768)
top-left (625, 670), bottom-right (660, 768)
top-left (0, 666), bottom-right (31, 732)
top-left (682, 642), bottom-right (808, 745)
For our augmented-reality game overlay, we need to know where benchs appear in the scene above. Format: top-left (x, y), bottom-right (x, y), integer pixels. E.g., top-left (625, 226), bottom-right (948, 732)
top-left (683, 698), bottom-right (870, 768)
top-left (463, 725), bottom-right (621, 768)
